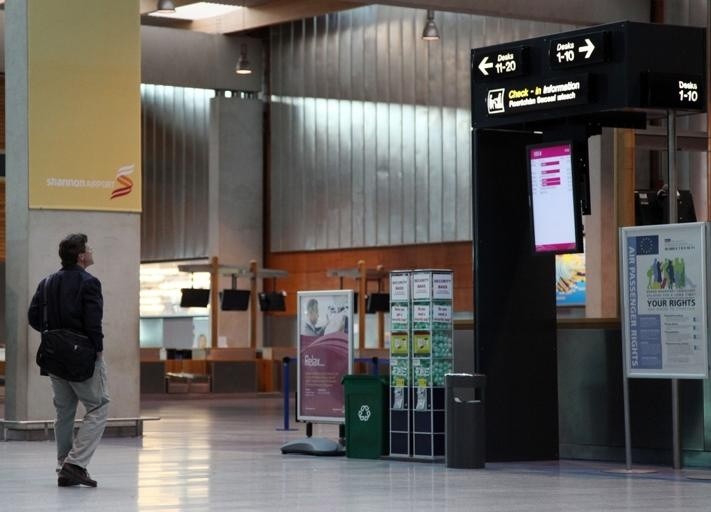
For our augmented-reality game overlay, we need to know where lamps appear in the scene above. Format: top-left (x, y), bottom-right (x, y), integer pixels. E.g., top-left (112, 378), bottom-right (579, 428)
top-left (422, 9), bottom-right (439, 41)
top-left (235, 34), bottom-right (255, 75)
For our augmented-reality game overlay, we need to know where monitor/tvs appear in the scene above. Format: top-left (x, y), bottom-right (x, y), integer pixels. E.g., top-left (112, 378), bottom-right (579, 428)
top-left (527, 139), bottom-right (585, 256)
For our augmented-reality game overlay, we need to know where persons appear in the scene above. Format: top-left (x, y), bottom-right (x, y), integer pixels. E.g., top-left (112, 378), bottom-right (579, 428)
top-left (302, 300), bottom-right (332, 336)
top-left (25, 234), bottom-right (109, 489)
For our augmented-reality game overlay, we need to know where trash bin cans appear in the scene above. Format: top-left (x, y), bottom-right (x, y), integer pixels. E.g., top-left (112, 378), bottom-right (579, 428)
top-left (446, 373), bottom-right (486, 469)
top-left (340, 374), bottom-right (387, 459)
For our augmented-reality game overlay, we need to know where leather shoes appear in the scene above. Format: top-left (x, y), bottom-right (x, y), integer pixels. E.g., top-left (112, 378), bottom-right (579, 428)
top-left (57, 469), bottom-right (82, 488)
top-left (59, 462), bottom-right (98, 490)
